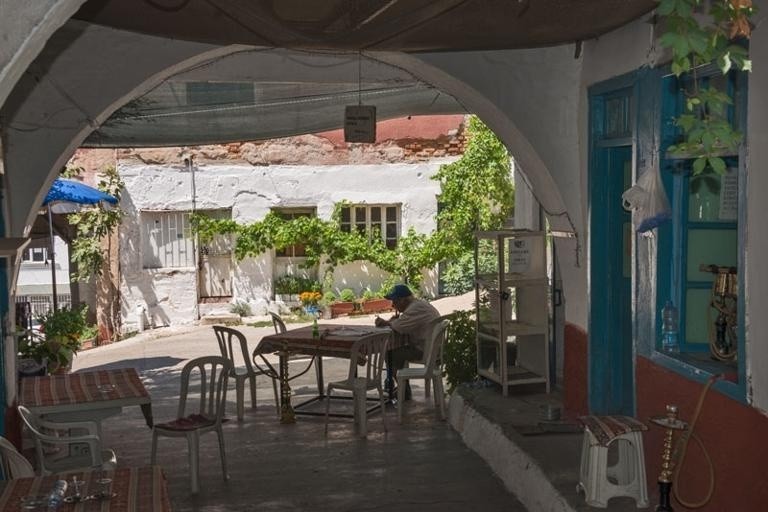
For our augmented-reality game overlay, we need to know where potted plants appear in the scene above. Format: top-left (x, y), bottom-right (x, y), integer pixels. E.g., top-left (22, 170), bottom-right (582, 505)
top-left (320, 287), bottom-right (394, 319)
top-left (16, 302), bottom-right (98, 379)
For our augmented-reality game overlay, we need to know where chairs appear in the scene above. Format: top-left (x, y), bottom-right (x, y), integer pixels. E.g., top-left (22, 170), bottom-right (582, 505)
top-left (151, 355), bottom-right (234, 494)
top-left (0, 436), bottom-right (36, 480)
top-left (213, 311), bottom-right (450, 438)
top-left (16, 404), bottom-right (102, 476)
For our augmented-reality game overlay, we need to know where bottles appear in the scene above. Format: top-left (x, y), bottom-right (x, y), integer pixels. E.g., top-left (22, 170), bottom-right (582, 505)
top-left (311, 318), bottom-right (319, 340)
top-left (660, 300), bottom-right (681, 356)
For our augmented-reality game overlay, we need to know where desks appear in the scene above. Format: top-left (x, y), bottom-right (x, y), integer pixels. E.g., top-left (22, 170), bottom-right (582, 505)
top-left (1, 464), bottom-right (172, 511)
top-left (17, 368), bottom-right (152, 456)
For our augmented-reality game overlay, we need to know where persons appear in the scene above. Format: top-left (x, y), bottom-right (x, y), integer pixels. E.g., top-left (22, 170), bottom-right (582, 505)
top-left (374, 283), bottom-right (445, 400)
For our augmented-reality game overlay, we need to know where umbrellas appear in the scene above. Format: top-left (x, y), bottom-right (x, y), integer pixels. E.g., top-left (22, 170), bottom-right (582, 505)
top-left (40, 178), bottom-right (120, 314)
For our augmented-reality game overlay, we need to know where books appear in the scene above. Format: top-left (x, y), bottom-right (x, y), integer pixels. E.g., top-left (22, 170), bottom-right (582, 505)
top-left (327, 329), bottom-right (378, 338)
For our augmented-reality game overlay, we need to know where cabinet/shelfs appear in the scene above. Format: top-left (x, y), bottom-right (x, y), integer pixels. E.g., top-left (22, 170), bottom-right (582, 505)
top-left (472, 229), bottom-right (551, 397)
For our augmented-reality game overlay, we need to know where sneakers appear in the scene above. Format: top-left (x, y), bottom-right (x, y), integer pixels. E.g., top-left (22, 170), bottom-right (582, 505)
top-left (390, 387), bottom-right (411, 399)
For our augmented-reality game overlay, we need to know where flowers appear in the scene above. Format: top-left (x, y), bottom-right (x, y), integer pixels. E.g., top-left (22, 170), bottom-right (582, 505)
top-left (300, 291), bottom-right (322, 309)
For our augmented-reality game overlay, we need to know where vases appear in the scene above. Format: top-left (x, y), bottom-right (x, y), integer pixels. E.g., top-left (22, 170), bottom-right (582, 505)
top-left (303, 306), bottom-right (319, 319)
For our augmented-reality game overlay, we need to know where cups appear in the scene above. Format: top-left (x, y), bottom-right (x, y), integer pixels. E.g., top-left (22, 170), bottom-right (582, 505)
top-left (666, 403), bottom-right (679, 424)
top-left (19, 447), bottom-right (118, 512)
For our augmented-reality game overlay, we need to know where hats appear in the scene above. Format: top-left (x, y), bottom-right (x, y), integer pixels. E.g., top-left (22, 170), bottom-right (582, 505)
top-left (383, 285), bottom-right (412, 300)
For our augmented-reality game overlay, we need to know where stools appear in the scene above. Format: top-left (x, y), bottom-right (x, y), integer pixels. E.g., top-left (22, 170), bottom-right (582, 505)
top-left (576, 416), bottom-right (650, 509)
